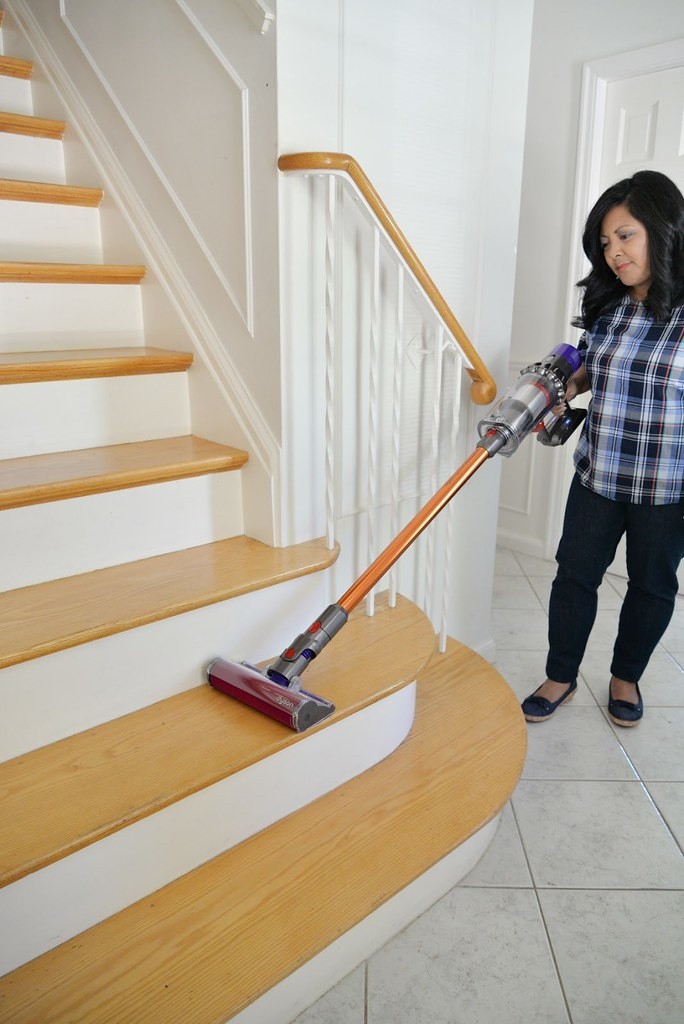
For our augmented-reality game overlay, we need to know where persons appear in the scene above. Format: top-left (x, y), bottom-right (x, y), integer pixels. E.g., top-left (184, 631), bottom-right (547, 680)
top-left (521, 172), bottom-right (684, 729)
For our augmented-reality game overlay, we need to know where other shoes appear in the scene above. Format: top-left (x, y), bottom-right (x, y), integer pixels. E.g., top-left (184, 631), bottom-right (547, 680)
top-left (521, 676), bottom-right (578, 721)
top-left (608, 676), bottom-right (643, 727)
